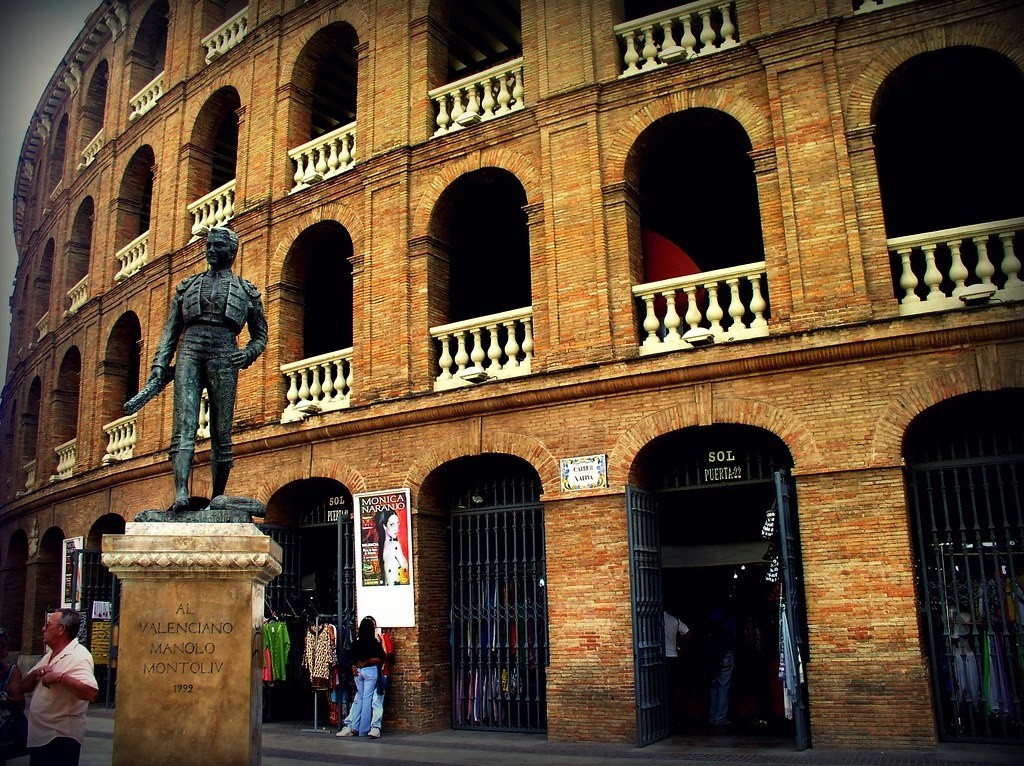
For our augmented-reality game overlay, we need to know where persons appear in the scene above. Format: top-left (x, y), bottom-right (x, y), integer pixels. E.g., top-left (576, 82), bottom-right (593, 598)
top-left (708, 610), bottom-right (740, 726)
top-left (374, 506), bottom-right (408, 586)
top-left (663, 610), bottom-right (693, 664)
top-left (148, 227), bottom-right (268, 509)
top-left (0, 608), bottom-right (100, 766)
top-left (336, 617), bottom-right (393, 738)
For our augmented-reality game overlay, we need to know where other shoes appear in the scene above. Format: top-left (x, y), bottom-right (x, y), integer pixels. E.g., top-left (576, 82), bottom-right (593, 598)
top-left (351, 729), bottom-right (359, 735)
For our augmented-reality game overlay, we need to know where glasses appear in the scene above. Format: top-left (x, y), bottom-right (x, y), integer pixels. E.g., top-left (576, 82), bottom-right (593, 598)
top-left (45, 622), bottom-right (62, 628)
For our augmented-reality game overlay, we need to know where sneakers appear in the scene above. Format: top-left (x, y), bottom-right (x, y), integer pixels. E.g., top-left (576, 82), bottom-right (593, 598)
top-left (709, 721), bottom-right (731, 725)
top-left (368, 728), bottom-right (380, 738)
top-left (336, 726), bottom-right (355, 737)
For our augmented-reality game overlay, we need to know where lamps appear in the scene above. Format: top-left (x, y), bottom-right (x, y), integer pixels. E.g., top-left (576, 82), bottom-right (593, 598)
top-left (49, 474), bottom-right (61, 482)
top-left (102, 454), bottom-right (118, 463)
top-left (62, 310), bottom-right (72, 319)
top-left (191, 224), bottom-right (209, 236)
top-left (114, 272), bottom-right (128, 282)
top-left (16, 492), bottom-right (25, 497)
top-left (27, 343), bottom-right (38, 350)
top-left (9, 370), bottom-right (15, 377)
top-left (460, 367), bottom-right (491, 384)
top-left (294, 399), bottom-right (322, 416)
top-left (682, 327), bottom-right (715, 347)
top-left (456, 111), bottom-right (482, 127)
top-left (302, 171), bottom-right (324, 185)
top-left (658, 46), bottom-right (688, 65)
top-left (959, 284), bottom-right (997, 307)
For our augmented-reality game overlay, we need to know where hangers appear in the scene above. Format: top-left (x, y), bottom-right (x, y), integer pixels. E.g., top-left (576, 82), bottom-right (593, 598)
top-left (267, 613), bottom-right (278, 623)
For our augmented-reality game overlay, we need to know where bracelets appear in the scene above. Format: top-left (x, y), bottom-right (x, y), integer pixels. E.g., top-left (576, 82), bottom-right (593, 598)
top-left (59, 673), bottom-right (66, 684)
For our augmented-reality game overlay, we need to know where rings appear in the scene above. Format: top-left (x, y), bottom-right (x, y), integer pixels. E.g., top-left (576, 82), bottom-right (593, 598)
top-left (41, 670), bottom-right (44, 673)
